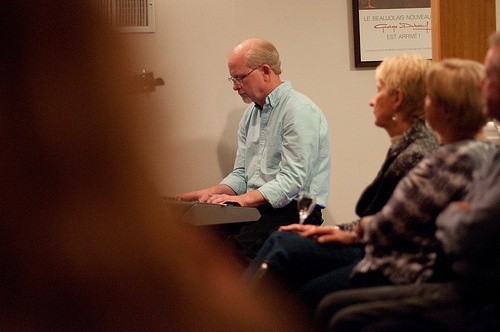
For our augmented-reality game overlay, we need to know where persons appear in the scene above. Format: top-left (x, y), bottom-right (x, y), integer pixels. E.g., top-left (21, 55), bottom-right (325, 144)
top-left (172, 39), bottom-right (329, 269)
top-left (247, 33), bottom-right (500, 332)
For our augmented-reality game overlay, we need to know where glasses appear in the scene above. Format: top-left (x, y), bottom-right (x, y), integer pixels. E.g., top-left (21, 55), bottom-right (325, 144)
top-left (228, 63), bottom-right (266, 85)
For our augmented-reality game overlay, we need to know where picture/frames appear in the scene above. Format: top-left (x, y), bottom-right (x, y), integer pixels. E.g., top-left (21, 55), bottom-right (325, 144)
top-left (352, 0), bottom-right (432, 67)
